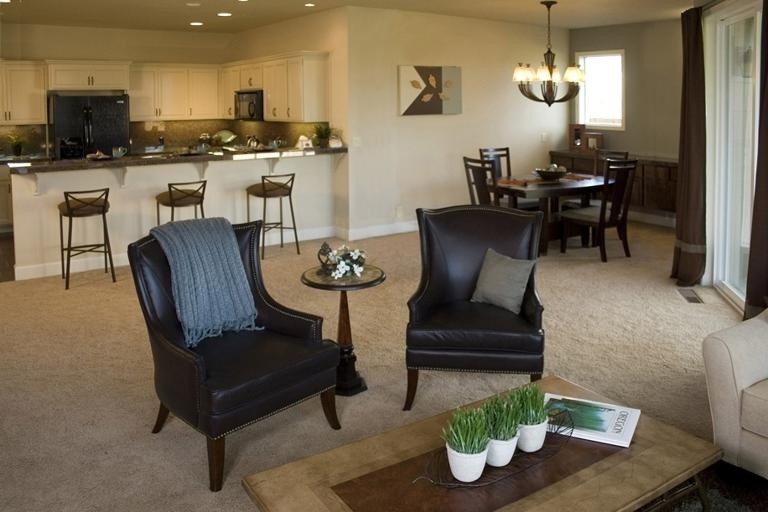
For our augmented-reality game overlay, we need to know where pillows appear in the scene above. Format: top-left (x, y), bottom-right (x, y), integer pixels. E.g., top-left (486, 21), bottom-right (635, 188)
top-left (470, 249), bottom-right (537, 314)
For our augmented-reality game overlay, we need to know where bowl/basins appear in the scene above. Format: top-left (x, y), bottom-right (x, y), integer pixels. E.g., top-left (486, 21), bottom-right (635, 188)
top-left (534, 168), bottom-right (568, 179)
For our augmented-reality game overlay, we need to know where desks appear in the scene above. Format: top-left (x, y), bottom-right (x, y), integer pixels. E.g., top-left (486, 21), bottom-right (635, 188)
top-left (486, 172), bottom-right (615, 258)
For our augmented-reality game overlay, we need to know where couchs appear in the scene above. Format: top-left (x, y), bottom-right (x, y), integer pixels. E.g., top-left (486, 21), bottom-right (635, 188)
top-left (699, 309), bottom-right (767, 480)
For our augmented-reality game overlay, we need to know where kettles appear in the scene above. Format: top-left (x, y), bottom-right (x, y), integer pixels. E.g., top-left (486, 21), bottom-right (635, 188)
top-left (246, 135), bottom-right (259, 148)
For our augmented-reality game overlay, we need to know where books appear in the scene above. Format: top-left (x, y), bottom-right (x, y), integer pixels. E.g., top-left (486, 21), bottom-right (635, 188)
top-left (542, 391), bottom-right (643, 448)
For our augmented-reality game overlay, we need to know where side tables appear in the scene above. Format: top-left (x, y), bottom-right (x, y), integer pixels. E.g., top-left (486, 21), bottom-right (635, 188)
top-left (301, 265), bottom-right (386, 397)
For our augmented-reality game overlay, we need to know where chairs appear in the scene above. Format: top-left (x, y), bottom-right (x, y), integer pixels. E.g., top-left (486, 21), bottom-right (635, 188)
top-left (127, 219), bottom-right (342, 492)
top-left (558, 159), bottom-right (640, 264)
top-left (402, 205), bottom-right (546, 410)
top-left (564, 152), bottom-right (627, 248)
top-left (246, 173), bottom-right (305, 260)
top-left (462, 156), bottom-right (538, 212)
top-left (154, 180), bottom-right (207, 226)
top-left (474, 147), bottom-right (542, 208)
top-left (58, 188), bottom-right (118, 291)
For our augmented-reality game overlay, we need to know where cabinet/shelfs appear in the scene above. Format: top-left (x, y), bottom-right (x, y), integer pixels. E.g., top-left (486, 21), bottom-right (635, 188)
top-left (1, 59), bottom-right (47, 126)
top-left (238, 58), bottom-right (263, 89)
top-left (48, 60), bottom-right (128, 90)
top-left (262, 51), bottom-right (330, 123)
top-left (548, 149), bottom-right (596, 174)
top-left (188, 62), bottom-right (220, 119)
top-left (218, 63), bottom-right (240, 121)
top-left (129, 62), bottom-right (189, 122)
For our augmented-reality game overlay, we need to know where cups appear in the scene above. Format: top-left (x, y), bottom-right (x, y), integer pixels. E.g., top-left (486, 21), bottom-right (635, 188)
top-left (112, 147), bottom-right (127, 159)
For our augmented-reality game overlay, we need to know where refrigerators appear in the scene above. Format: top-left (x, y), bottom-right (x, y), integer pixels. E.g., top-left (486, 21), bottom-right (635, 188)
top-left (48, 90), bottom-right (132, 159)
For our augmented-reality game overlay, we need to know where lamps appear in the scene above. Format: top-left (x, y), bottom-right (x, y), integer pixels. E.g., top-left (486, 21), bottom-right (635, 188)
top-left (188, 21), bottom-right (205, 28)
top-left (305, 4), bottom-right (315, 7)
top-left (511, 1), bottom-right (586, 108)
top-left (216, 12), bottom-right (232, 17)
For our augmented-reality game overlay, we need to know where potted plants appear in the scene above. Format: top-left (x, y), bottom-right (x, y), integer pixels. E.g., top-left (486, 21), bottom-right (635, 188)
top-left (312, 124), bottom-right (331, 149)
top-left (441, 409), bottom-right (490, 481)
top-left (507, 383), bottom-right (549, 453)
top-left (477, 395), bottom-right (520, 468)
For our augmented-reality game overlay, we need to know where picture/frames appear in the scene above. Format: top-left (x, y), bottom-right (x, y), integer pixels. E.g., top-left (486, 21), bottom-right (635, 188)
top-left (399, 64), bottom-right (462, 116)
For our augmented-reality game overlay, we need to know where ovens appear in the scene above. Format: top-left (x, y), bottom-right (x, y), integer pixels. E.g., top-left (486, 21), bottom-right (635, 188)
top-left (233, 91), bottom-right (263, 121)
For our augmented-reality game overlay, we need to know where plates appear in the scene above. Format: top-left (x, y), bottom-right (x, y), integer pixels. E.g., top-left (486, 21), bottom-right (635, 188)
top-left (86, 154), bottom-right (108, 159)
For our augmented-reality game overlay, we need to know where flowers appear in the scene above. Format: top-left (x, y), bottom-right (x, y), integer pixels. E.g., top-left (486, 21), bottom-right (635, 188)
top-left (328, 244), bottom-right (368, 280)
